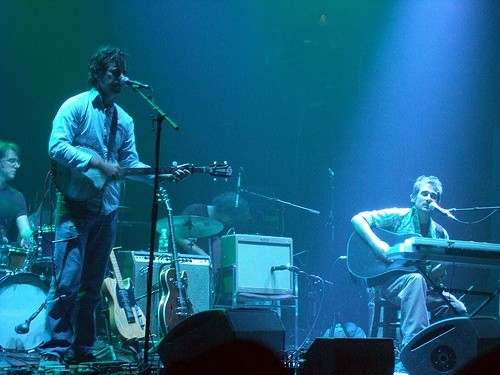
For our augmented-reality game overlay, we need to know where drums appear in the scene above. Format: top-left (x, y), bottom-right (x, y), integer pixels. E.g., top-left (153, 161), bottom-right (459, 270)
top-left (29, 225), bottom-right (56, 276)
top-left (0, 270), bottom-right (52, 353)
top-left (0, 244), bottom-right (33, 274)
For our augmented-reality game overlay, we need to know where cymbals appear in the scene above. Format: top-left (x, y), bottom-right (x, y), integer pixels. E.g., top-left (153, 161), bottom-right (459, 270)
top-left (156, 215), bottom-right (224, 238)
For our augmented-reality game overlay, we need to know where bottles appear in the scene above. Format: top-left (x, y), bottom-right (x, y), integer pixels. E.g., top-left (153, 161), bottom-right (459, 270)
top-left (158, 229), bottom-right (168, 252)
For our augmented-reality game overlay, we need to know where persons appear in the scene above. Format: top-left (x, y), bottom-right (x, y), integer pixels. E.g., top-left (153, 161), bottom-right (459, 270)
top-left (39, 45), bottom-right (192, 375)
top-left (0, 140), bottom-right (37, 248)
top-left (175, 190), bottom-right (249, 255)
top-left (351, 175), bottom-right (468, 375)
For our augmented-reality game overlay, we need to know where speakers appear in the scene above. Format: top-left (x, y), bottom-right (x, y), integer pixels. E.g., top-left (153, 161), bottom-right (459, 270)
top-left (397, 315), bottom-right (500, 375)
top-left (304, 337), bottom-right (394, 375)
top-left (117, 250), bottom-right (209, 345)
top-left (158, 308), bottom-right (285, 362)
top-left (220, 233), bottom-right (294, 296)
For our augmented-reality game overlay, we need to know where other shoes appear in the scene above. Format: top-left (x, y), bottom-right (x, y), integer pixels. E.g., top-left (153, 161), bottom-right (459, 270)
top-left (38, 354), bottom-right (60, 374)
top-left (63, 355), bottom-right (99, 366)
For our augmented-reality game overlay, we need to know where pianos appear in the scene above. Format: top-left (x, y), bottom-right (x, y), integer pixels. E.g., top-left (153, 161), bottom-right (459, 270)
top-left (385, 236), bottom-right (500, 320)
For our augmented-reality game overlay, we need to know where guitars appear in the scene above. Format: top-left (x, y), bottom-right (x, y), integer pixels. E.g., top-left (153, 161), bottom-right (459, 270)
top-left (347, 226), bottom-right (424, 288)
top-left (156, 186), bottom-right (196, 336)
top-left (101, 248), bottom-right (146, 341)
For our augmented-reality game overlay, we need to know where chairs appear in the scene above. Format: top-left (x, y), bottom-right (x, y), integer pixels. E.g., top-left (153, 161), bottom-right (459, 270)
top-left (371, 284), bottom-right (401, 337)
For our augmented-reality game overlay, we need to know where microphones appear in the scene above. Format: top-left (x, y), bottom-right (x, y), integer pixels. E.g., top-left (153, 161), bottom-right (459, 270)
top-left (234, 171), bottom-right (241, 208)
top-left (120, 76), bottom-right (151, 89)
top-left (271, 264), bottom-right (289, 271)
top-left (429, 202), bottom-right (457, 219)
top-left (14, 322), bottom-right (30, 333)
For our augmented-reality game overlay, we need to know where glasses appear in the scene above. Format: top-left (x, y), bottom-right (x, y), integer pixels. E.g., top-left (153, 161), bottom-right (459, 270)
top-left (0, 159), bottom-right (22, 166)
